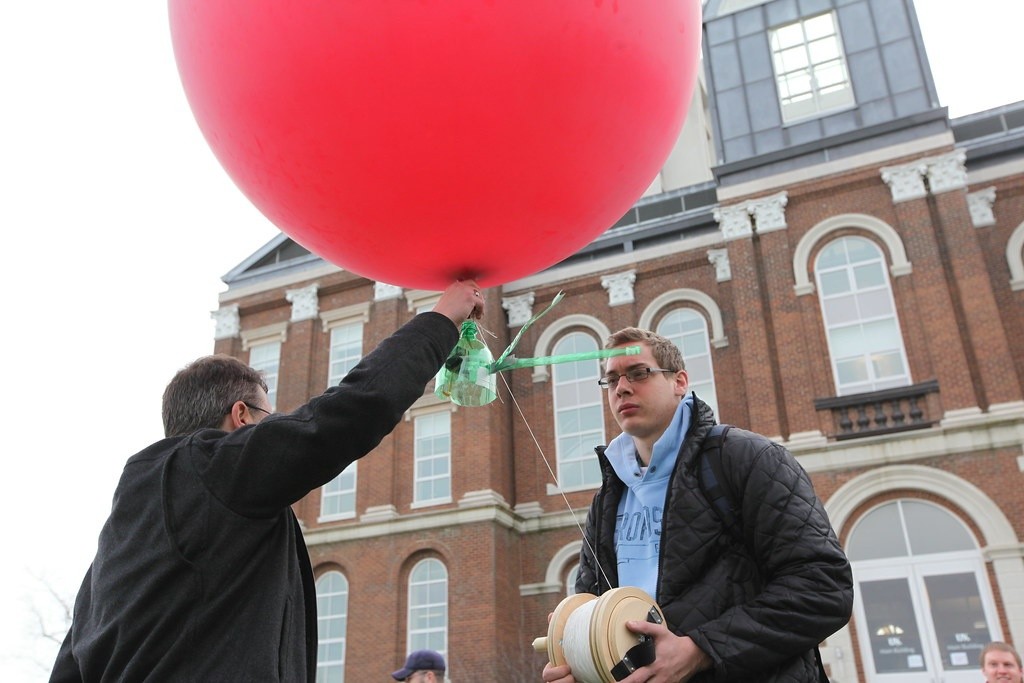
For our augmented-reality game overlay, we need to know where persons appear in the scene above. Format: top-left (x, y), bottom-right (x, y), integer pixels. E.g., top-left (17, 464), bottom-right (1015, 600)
top-left (391, 650), bottom-right (446, 683)
top-left (542, 327), bottom-right (854, 683)
top-left (980, 641), bottom-right (1024, 683)
top-left (50, 281), bottom-right (484, 683)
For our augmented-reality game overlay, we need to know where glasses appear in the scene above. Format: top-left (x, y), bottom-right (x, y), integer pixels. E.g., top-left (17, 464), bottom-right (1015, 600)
top-left (598, 368), bottom-right (674, 389)
top-left (405, 671), bottom-right (427, 683)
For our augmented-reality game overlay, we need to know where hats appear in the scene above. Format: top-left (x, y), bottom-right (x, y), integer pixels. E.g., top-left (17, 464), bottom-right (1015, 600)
top-left (391, 650), bottom-right (446, 681)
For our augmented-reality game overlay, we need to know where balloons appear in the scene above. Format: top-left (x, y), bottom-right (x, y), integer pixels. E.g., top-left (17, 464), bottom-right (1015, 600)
top-left (166, 0), bottom-right (700, 291)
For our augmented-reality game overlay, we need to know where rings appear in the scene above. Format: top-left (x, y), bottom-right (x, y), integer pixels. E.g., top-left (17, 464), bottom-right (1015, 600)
top-left (475, 290), bottom-right (480, 296)
